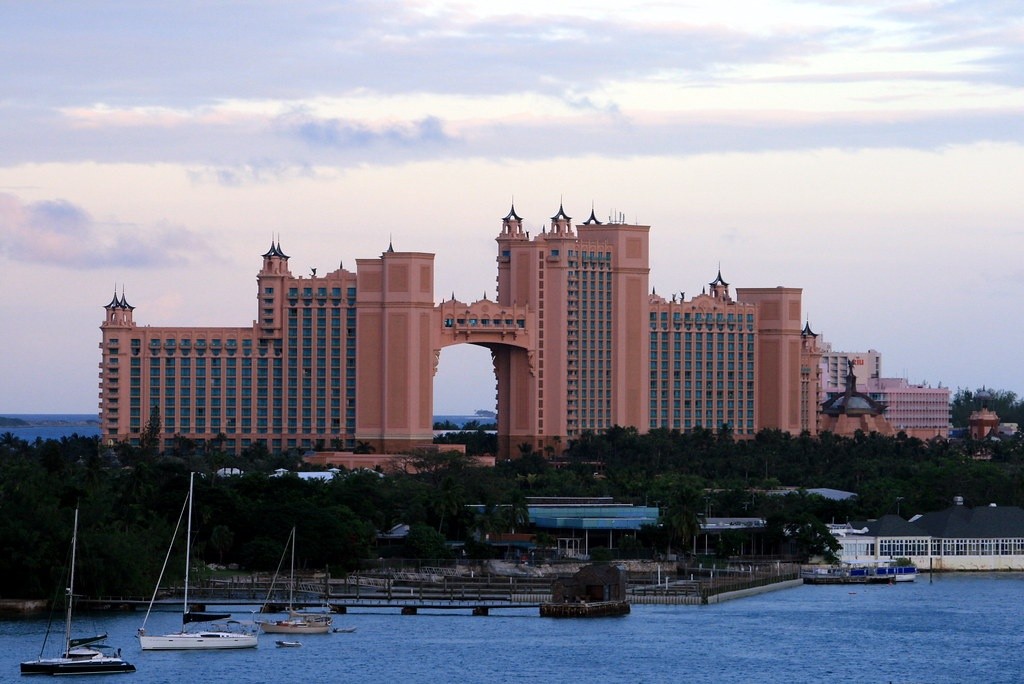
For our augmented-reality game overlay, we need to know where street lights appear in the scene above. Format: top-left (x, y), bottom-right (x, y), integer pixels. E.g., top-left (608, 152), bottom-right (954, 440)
top-left (896, 496), bottom-right (905, 517)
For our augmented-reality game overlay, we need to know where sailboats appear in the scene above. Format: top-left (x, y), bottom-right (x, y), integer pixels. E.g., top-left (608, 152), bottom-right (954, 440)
top-left (134, 472), bottom-right (259, 650)
top-left (19, 500), bottom-right (137, 676)
top-left (254, 526), bottom-right (334, 634)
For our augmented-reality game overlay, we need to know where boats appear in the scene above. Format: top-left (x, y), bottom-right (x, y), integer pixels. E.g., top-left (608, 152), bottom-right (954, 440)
top-left (333, 626), bottom-right (358, 632)
top-left (275, 640), bottom-right (303, 647)
top-left (799, 559), bottom-right (919, 583)
